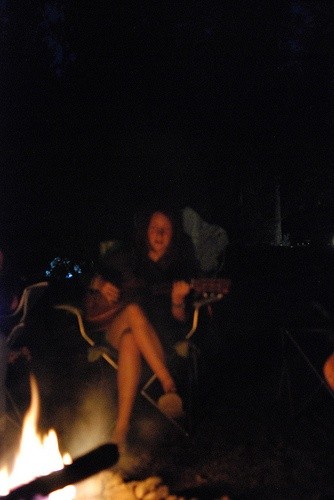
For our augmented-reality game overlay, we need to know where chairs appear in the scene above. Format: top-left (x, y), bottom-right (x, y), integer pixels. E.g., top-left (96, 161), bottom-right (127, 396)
top-left (51, 237), bottom-right (230, 445)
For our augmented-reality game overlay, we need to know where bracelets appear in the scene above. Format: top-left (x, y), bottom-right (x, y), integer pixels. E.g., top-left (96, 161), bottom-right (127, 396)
top-left (172, 302), bottom-right (185, 307)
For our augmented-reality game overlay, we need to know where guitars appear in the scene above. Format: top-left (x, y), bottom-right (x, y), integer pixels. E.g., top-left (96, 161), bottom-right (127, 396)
top-left (82, 275), bottom-right (231, 331)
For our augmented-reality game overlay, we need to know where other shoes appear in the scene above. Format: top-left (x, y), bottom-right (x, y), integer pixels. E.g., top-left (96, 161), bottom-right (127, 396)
top-left (158, 391), bottom-right (187, 421)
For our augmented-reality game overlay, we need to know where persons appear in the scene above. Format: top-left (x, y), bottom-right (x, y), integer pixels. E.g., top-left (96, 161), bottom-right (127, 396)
top-left (87, 209), bottom-right (189, 454)
